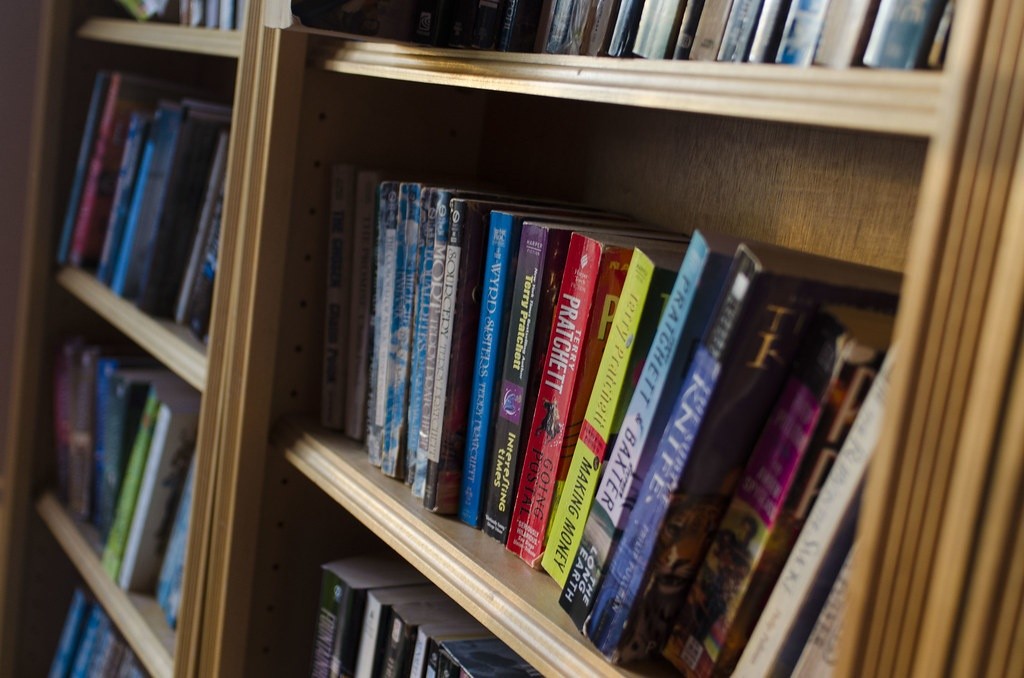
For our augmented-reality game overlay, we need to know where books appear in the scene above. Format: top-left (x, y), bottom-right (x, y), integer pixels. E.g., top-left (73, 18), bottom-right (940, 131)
top-left (45, 582), bottom-right (152, 678)
top-left (52, 60), bottom-right (233, 341)
top-left (306, 155), bottom-right (897, 678)
top-left (53, 332), bottom-right (202, 632)
top-left (116, 0), bottom-right (960, 71)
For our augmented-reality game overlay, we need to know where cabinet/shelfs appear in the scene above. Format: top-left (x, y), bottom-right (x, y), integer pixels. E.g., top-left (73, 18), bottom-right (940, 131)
top-left (0, 0), bottom-right (1024, 678)
